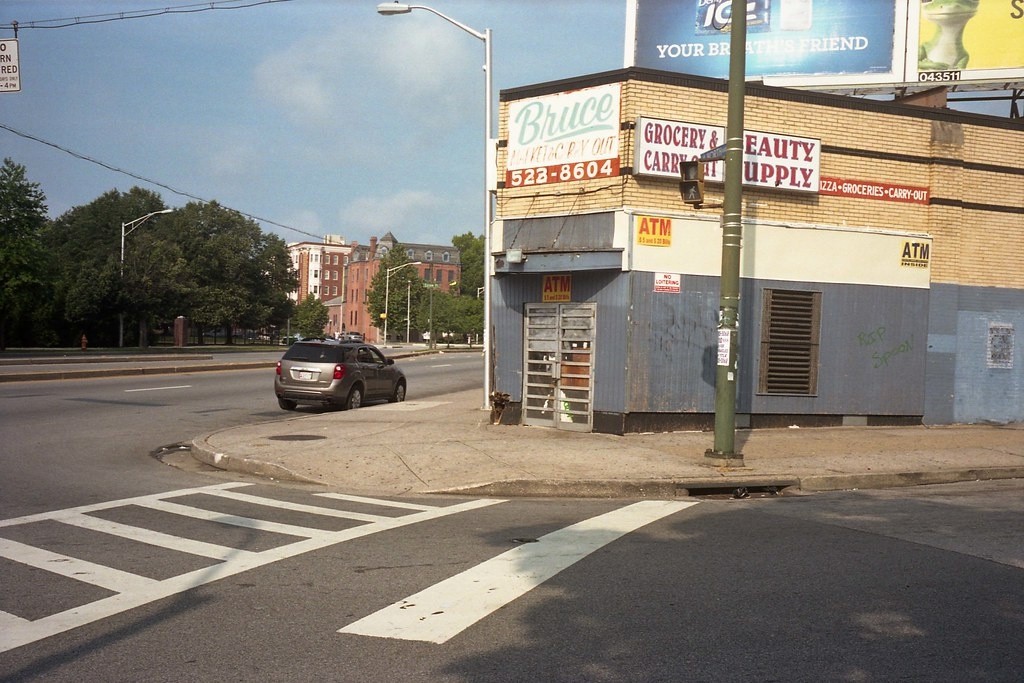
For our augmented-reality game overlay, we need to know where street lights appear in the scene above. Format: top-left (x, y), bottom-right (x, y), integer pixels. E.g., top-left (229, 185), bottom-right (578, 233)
top-left (375, 0), bottom-right (497, 411)
top-left (383, 262), bottom-right (422, 345)
top-left (118, 208), bottom-right (177, 348)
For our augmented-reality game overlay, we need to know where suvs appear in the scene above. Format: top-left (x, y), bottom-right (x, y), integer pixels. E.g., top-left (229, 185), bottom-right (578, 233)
top-left (274, 337), bottom-right (407, 413)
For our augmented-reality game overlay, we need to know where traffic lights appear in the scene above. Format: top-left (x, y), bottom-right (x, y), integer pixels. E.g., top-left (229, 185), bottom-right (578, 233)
top-left (680, 163), bottom-right (702, 201)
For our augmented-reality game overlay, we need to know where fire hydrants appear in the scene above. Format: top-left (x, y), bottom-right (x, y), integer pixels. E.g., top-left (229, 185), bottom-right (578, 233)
top-left (81, 334), bottom-right (89, 351)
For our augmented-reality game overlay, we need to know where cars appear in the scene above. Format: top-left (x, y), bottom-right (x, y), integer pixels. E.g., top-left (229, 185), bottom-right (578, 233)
top-left (340, 331), bottom-right (364, 340)
